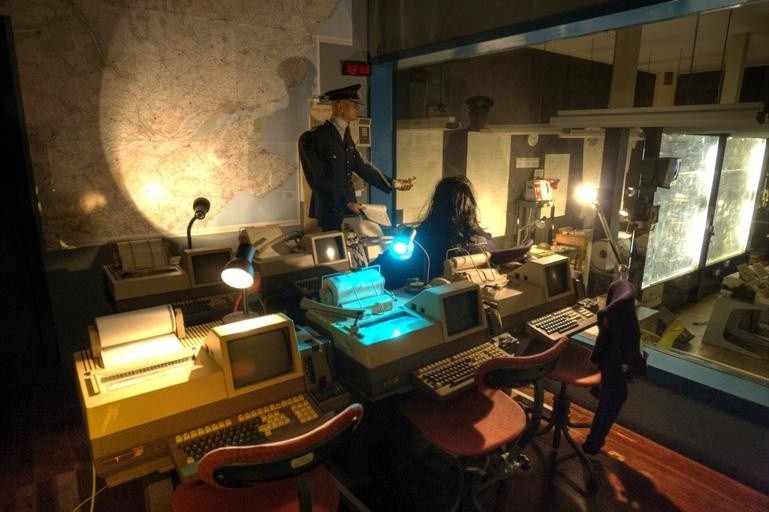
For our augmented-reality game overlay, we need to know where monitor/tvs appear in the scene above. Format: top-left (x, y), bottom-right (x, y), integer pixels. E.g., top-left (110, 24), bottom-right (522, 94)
top-left (654, 157), bottom-right (682, 189)
top-left (406, 280), bottom-right (489, 344)
top-left (507, 254), bottom-right (575, 303)
top-left (203, 313), bottom-right (305, 397)
top-left (179, 247), bottom-right (233, 290)
top-left (299, 229), bottom-right (349, 267)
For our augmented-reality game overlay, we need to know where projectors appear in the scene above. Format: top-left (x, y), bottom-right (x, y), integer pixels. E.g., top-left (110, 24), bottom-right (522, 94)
top-left (408, 115), bottom-right (462, 133)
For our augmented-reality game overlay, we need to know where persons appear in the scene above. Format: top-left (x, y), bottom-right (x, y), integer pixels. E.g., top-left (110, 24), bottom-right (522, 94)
top-left (297, 82), bottom-right (417, 231)
top-left (370, 176), bottom-right (495, 291)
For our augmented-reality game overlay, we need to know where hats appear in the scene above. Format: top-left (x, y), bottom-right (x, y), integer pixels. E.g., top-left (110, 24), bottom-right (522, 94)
top-left (324, 83), bottom-right (365, 105)
top-left (466, 95), bottom-right (495, 113)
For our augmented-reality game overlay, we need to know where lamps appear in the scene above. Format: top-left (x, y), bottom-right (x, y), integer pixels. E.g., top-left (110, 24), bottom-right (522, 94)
top-left (187, 197), bottom-right (210, 248)
top-left (548, 100), bottom-right (766, 131)
top-left (558, 129), bottom-right (606, 139)
top-left (477, 123), bottom-right (571, 135)
top-left (221, 242), bottom-right (261, 323)
top-left (396, 115), bottom-right (464, 132)
top-left (391, 228), bottom-right (431, 286)
top-left (578, 185), bottom-right (636, 271)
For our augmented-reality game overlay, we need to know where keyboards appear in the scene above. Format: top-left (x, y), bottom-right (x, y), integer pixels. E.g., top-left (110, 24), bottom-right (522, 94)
top-left (170, 292), bottom-right (234, 316)
top-left (410, 341), bottom-right (513, 400)
top-left (524, 303), bottom-right (599, 348)
top-left (166, 393), bottom-right (327, 483)
top-left (293, 276), bottom-right (321, 296)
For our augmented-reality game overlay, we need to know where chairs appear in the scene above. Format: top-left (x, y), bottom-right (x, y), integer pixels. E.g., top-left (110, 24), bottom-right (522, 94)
top-left (398, 336), bottom-right (569, 504)
top-left (522, 280), bottom-right (646, 491)
top-left (170, 404), bottom-right (363, 512)
